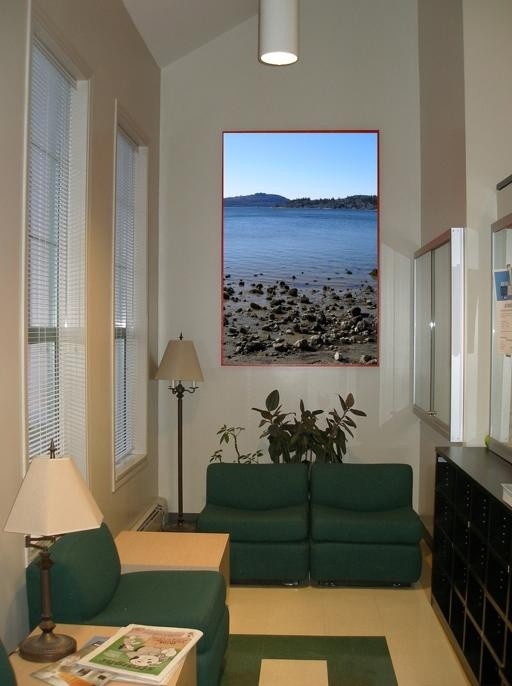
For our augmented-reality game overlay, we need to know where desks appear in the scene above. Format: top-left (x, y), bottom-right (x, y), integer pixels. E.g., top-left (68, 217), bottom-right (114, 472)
top-left (112, 525), bottom-right (230, 609)
top-left (2, 623), bottom-right (201, 685)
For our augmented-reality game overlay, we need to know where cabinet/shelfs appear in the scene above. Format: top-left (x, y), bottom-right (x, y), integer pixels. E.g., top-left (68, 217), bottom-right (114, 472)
top-left (430, 446), bottom-right (512, 685)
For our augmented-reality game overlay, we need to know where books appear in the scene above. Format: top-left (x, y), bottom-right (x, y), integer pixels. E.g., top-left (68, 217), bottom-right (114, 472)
top-left (497, 481), bottom-right (512, 509)
top-left (27, 622), bottom-right (205, 686)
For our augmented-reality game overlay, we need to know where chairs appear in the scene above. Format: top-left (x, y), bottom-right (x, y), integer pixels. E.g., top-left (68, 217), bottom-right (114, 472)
top-left (26, 524), bottom-right (235, 686)
top-left (201, 461), bottom-right (310, 580)
top-left (310, 463), bottom-right (426, 586)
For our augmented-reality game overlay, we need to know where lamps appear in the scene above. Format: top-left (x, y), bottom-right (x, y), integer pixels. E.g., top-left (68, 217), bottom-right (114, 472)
top-left (256, 2), bottom-right (298, 64)
top-left (9, 439), bottom-right (108, 662)
top-left (153, 333), bottom-right (206, 533)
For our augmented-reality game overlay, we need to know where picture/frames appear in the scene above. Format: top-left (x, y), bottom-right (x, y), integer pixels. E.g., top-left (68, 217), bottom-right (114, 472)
top-left (219, 127), bottom-right (383, 370)
